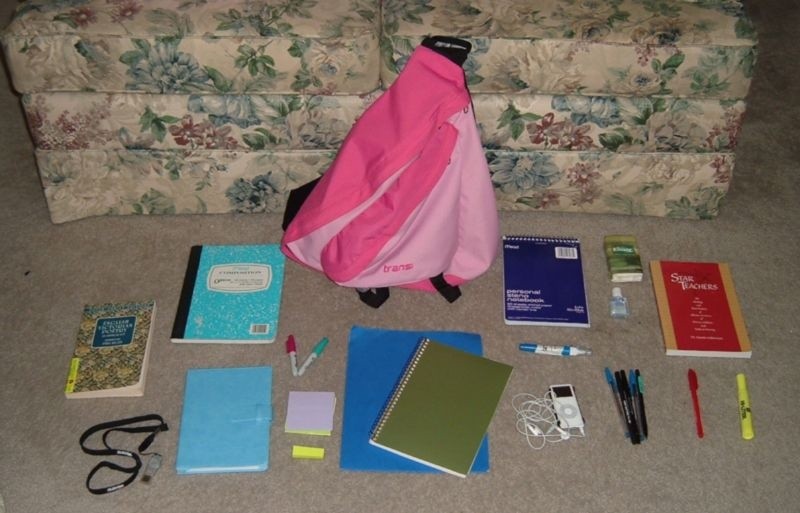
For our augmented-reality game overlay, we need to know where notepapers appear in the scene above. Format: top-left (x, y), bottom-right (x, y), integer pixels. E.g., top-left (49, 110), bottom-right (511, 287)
top-left (291, 444), bottom-right (324, 460)
top-left (284, 391), bottom-right (337, 436)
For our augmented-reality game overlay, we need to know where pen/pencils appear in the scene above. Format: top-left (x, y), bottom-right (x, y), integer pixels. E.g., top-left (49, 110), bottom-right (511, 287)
top-left (286, 335), bottom-right (298, 376)
top-left (298, 337), bottom-right (330, 376)
top-left (604, 367), bottom-right (648, 445)
top-left (688, 368), bottom-right (705, 438)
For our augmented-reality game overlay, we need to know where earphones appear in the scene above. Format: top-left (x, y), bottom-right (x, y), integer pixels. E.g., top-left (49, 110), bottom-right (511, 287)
top-left (527, 424), bottom-right (541, 436)
top-left (557, 427), bottom-right (570, 440)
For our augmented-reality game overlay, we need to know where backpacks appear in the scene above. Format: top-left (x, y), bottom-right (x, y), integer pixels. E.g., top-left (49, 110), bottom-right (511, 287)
top-left (280, 33), bottom-right (499, 310)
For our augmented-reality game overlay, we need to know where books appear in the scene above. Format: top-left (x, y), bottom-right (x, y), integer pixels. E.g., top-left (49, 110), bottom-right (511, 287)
top-left (175, 363), bottom-right (273, 476)
top-left (64, 297), bottom-right (158, 400)
top-left (501, 233), bottom-right (592, 329)
top-left (168, 242), bottom-right (286, 345)
top-left (367, 335), bottom-right (517, 481)
top-left (648, 259), bottom-right (754, 360)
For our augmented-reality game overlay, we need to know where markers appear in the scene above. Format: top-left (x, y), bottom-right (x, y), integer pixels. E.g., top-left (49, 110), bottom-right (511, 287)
top-left (736, 374), bottom-right (754, 440)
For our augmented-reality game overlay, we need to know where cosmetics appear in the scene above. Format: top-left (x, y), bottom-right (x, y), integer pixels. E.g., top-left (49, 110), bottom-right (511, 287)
top-left (608, 286), bottom-right (630, 320)
